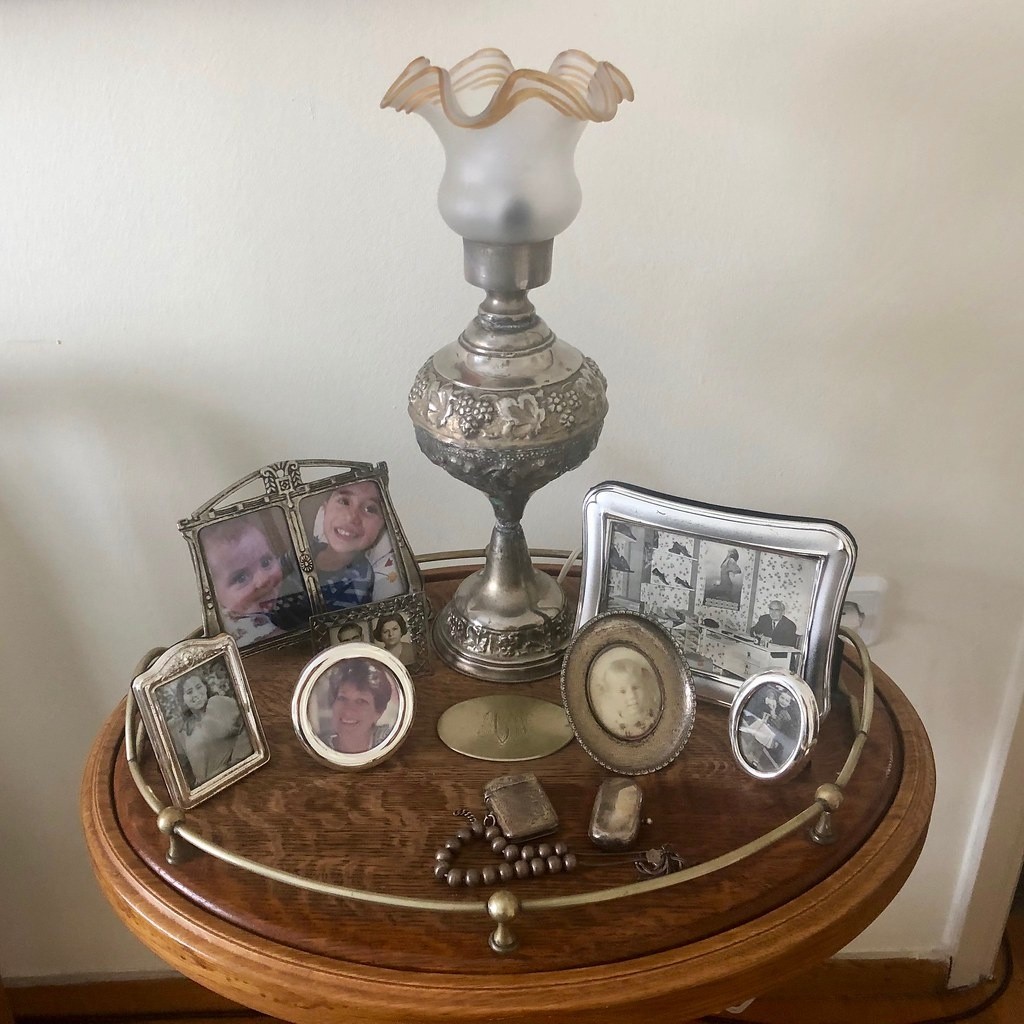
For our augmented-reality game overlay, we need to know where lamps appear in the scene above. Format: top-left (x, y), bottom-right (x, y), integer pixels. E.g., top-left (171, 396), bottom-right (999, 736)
top-left (377, 46), bottom-right (638, 686)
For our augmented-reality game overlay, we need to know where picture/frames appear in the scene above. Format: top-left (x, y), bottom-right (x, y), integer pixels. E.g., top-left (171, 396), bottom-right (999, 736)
top-left (309, 591), bottom-right (434, 681)
top-left (727, 665), bottom-right (819, 787)
top-left (560, 609), bottom-right (698, 775)
top-left (290, 640), bottom-right (419, 773)
top-left (566, 480), bottom-right (857, 727)
top-left (177, 458), bottom-right (426, 656)
top-left (131, 632), bottom-right (272, 810)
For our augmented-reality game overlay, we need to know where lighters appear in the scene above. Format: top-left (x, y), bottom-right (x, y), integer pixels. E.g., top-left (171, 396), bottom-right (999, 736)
top-left (483, 774), bottom-right (559, 843)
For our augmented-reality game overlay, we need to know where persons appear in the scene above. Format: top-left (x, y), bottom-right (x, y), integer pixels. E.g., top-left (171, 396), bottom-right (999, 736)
top-left (319, 663), bottom-right (394, 753)
top-left (745, 689), bottom-right (795, 769)
top-left (602, 659), bottom-right (658, 739)
top-left (309, 482), bottom-right (386, 613)
top-left (712, 548), bottom-right (741, 594)
top-left (338, 624), bottom-right (365, 645)
top-left (751, 601), bottom-right (796, 673)
top-left (176, 672), bottom-right (246, 787)
top-left (200, 520), bottom-right (301, 648)
top-left (373, 613), bottom-right (415, 664)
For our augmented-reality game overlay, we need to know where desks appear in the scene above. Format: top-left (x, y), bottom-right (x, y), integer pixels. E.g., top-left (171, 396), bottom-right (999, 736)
top-left (84, 541), bottom-right (941, 1024)
